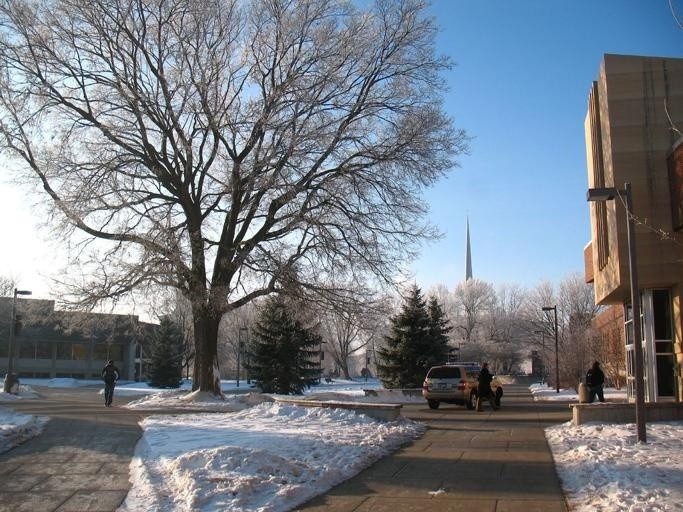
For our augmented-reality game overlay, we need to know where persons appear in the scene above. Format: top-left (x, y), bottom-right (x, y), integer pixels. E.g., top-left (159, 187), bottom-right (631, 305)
top-left (475, 362), bottom-right (497, 412)
top-left (585, 362), bottom-right (604, 403)
top-left (101, 360), bottom-right (120, 407)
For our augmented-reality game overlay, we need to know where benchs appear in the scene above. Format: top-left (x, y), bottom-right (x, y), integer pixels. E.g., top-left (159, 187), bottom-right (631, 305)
top-left (569, 402), bottom-right (683, 424)
top-left (276, 398), bottom-right (403, 422)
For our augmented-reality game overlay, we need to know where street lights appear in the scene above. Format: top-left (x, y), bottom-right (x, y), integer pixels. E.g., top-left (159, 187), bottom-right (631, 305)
top-left (586, 182), bottom-right (646, 442)
top-left (319, 341), bottom-right (328, 382)
top-left (542, 305), bottom-right (559, 392)
top-left (237, 326), bottom-right (249, 387)
top-left (534, 331), bottom-right (545, 380)
top-left (365, 349), bottom-right (372, 381)
top-left (8, 288), bottom-right (33, 373)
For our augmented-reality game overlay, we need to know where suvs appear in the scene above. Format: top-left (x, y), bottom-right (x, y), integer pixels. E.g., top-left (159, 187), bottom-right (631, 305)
top-left (422, 362), bottom-right (503, 410)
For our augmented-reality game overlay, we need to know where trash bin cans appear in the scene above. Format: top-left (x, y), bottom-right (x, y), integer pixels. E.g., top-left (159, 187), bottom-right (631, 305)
top-left (579, 382), bottom-right (591, 403)
